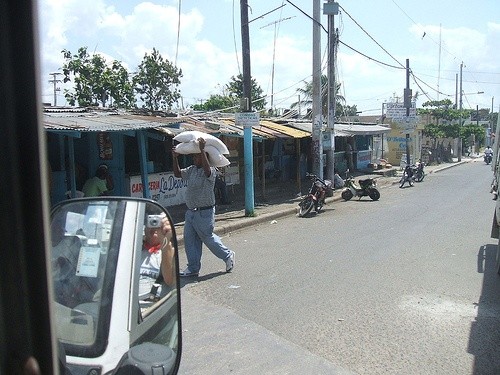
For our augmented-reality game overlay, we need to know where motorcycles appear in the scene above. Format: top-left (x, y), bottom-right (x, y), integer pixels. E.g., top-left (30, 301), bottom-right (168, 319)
top-left (298, 172), bottom-right (336, 217)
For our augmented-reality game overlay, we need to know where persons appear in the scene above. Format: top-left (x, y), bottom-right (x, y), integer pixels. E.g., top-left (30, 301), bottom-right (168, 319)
top-left (138, 211), bottom-right (175, 314)
top-left (82, 163), bottom-right (115, 197)
top-left (484, 145), bottom-right (493, 158)
top-left (172, 136), bottom-right (235, 277)
top-left (345, 138), bottom-right (358, 173)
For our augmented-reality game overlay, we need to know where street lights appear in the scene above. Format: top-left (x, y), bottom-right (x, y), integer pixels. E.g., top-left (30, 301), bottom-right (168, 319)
top-left (458, 89), bottom-right (485, 162)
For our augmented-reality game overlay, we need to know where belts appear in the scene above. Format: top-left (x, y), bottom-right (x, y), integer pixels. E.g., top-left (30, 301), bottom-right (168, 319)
top-left (194, 205), bottom-right (215, 211)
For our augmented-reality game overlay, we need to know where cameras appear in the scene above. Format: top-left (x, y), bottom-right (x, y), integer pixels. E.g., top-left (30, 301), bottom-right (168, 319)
top-left (146, 215), bottom-right (163, 228)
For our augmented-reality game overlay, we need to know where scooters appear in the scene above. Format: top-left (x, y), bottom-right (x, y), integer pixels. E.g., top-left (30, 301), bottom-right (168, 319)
top-left (483, 152), bottom-right (493, 165)
top-left (341, 168), bottom-right (380, 201)
top-left (398, 159), bottom-right (425, 188)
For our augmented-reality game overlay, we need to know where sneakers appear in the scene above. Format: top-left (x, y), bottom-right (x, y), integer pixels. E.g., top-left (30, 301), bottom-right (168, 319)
top-left (179, 267), bottom-right (199, 276)
top-left (225, 251), bottom-right (235, 273)
